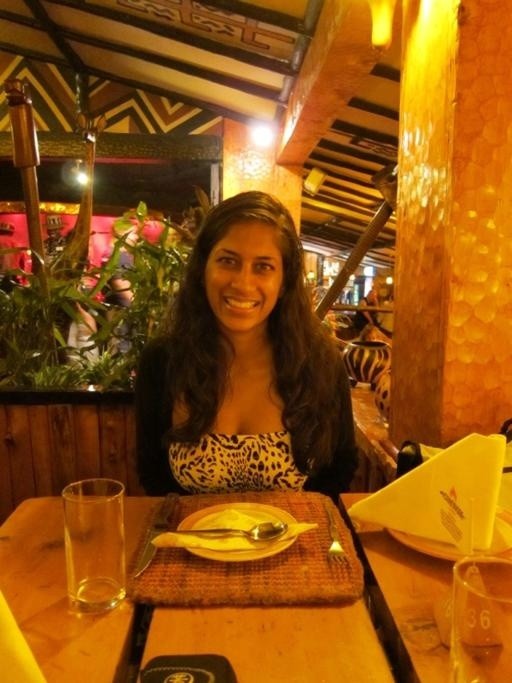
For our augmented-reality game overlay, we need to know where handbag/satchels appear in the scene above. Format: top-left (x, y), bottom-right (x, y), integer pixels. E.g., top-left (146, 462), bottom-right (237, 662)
top-left (397, 418), bottom-right (512, 495)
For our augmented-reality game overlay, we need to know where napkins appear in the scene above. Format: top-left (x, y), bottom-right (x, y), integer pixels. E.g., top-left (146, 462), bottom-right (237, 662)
top-left (150, 508), bottom-right (319, 552)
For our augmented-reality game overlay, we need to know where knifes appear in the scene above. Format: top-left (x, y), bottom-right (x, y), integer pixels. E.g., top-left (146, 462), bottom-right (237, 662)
top-left (134, 491), bottom-right (180, 579)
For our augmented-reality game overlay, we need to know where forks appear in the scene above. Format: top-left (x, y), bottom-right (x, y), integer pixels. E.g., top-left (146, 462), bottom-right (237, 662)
top-left (322, 499), bottom-right (351, 572)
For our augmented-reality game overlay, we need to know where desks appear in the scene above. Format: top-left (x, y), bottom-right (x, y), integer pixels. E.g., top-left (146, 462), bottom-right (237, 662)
top-left (337, 493), bottom-right (511, 676)
top-left (1, 487), bottom-right (395, 677)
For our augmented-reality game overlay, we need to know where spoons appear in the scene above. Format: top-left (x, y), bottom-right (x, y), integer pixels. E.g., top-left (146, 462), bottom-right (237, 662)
top-left (150, 520), bottom-right (290, 543)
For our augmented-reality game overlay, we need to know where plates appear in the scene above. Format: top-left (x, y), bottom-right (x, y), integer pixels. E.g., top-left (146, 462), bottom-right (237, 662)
top-left (176, 501), bottom-right (299, 563)
top-left (381, 510), bottom-right (512, 567)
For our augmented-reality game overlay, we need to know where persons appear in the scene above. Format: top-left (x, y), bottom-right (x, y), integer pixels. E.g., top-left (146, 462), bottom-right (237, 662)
top-left (134, 191), bottom-right (358, 496)
top-left (101, 215), bottom-right (161, 357)
top-left (43, 215), bottom-right (66, 256)
top-left (311, 270), bottom-right (380, 331)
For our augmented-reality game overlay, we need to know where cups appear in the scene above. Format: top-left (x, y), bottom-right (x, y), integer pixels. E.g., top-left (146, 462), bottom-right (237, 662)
top-left (450, 552), bottom-right (512, 683)
top-left (62, 476), bottom-right (129, 614)
